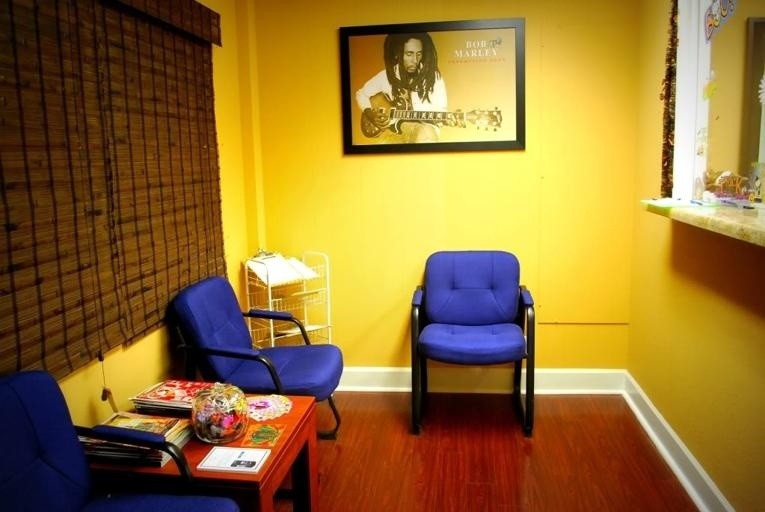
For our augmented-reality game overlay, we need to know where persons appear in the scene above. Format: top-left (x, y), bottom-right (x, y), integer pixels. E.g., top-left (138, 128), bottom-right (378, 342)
top-left (355, 32), bottom-right (448, 144)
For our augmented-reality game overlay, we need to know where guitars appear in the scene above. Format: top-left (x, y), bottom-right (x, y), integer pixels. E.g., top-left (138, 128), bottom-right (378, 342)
top-left (361, 92), bottom-right (502, 137)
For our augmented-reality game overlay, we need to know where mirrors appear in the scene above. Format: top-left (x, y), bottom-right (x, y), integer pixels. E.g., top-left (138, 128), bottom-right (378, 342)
top-left (705, 0), bottom-right (765, 211)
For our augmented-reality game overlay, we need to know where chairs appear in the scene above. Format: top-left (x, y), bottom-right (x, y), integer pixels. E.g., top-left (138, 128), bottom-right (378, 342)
top-left (410, 250), bottom-right (535, 436)
top-left (0, 370), bottom-right (242, 512)
top-left (166, 276), bottom-right (344, 438)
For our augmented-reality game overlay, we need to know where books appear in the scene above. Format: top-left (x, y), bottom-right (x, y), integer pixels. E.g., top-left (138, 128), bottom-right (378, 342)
top-left (196, 446), bottom-right (272, 475)
top-left (77, 377), bottom-right (215, 467)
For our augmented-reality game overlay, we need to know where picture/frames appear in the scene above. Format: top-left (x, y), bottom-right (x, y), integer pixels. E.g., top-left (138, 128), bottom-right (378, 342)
top-left (338, 16), bottom-right (525, 153)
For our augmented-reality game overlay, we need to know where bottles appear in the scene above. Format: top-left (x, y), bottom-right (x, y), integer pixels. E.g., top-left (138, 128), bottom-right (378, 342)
top-left (189, 382), bottom-right (251, 443)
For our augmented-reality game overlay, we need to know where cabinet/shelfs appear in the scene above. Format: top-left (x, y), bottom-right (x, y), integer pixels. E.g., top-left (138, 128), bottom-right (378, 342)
top-left (245, 250), bottom-right (332, 349)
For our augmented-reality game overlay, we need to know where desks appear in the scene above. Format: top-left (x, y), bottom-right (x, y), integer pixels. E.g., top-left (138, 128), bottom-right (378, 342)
top-left (88, 394), bottom-right (320, 512)
